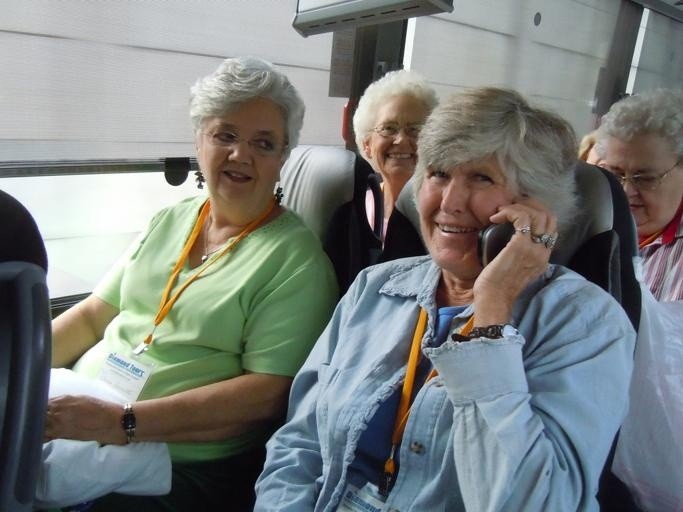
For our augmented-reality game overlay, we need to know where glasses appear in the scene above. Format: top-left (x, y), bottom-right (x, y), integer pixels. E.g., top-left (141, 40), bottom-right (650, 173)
top-left (612, 165), bottom-right (675, 189)
top-left (369, 122), bottom-right (424, 137)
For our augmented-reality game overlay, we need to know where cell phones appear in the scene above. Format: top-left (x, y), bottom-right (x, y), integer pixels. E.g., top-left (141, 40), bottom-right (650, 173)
top-left (478, 220), bottom-right (514, 269)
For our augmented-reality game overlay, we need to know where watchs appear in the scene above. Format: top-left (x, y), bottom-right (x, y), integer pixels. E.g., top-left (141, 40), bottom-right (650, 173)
top-left (121, 404), bottom-right (135, 445)
top-left (466, 323), bottom-right (524, 339)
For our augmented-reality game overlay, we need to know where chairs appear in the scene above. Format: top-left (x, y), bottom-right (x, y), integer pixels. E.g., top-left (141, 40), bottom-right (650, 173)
top-left (273, 145), bottom-right (384, 299)
top-left (383, 157), bottom-right (646, 511)
top-left (0, 191), bottom-right (52, 511)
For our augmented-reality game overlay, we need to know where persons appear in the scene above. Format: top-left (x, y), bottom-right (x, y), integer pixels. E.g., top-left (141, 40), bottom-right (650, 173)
top-left (252, 85), bottom-right (636, 512)
top-left (41, 54), bottom-right (340, 512)
top-left (596, 89), bottom-right (683, 512)
top-left (323, 68), bottom-right (440, 297)
top-left (576, 129), bottom-right (611, 172)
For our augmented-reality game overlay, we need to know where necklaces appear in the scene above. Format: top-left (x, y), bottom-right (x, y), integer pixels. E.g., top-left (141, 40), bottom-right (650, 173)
top-left (198, 208), bottom-right (234, 263)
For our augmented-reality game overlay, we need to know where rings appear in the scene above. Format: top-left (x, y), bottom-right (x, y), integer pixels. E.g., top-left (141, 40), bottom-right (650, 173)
top-left (533, 231), bottom-right (549, 244)
top-left (546, 237), bottom-right (556, 247)
top-left (515, 226), bottom-right (531, 234)
